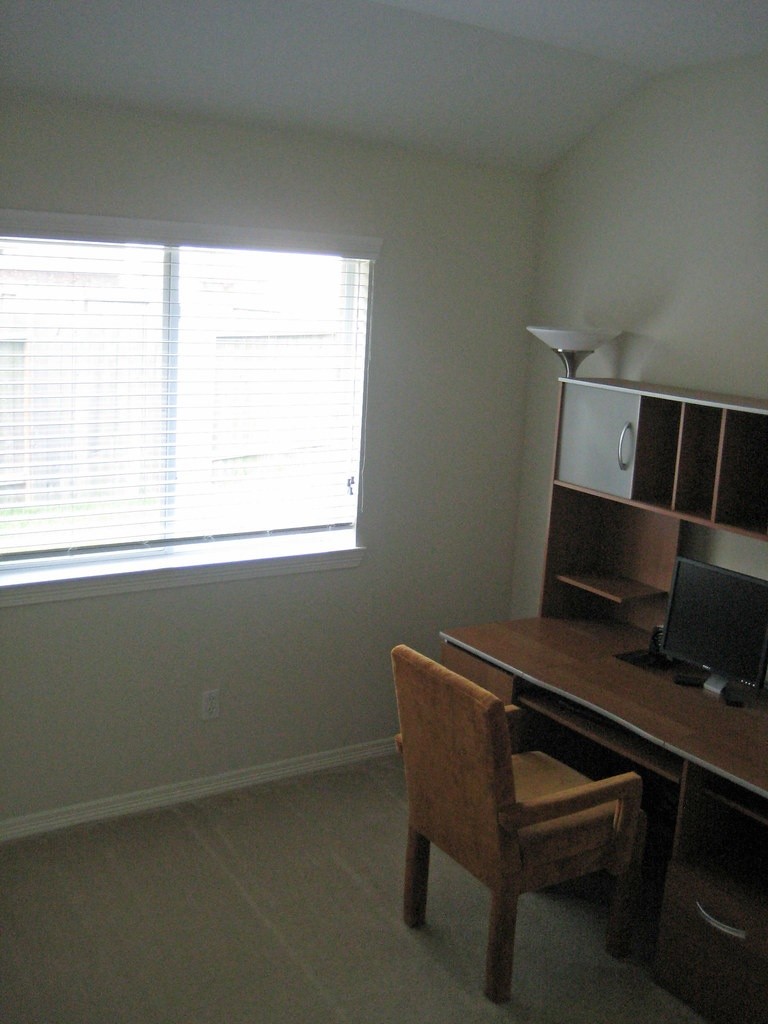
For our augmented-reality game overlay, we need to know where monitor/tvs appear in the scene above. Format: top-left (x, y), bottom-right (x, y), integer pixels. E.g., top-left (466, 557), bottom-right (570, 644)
top-left (658, 557), bottom-right (768, 708)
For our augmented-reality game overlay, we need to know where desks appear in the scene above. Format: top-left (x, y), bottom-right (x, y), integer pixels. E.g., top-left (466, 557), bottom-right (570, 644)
top-left (438, 616), bottom-right (768, 1024)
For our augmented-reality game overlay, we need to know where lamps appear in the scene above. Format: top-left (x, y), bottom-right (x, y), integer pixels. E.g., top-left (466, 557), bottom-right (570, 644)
top-left (527, 326), bottom-right (622, 377)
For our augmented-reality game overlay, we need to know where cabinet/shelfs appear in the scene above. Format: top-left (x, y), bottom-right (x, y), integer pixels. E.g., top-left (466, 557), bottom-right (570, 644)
top-left (539, 377), bottom-right (768, 635)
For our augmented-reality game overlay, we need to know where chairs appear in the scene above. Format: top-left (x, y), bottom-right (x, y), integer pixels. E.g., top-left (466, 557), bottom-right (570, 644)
top-left (391, 643), bottom-right (649, 1006)
